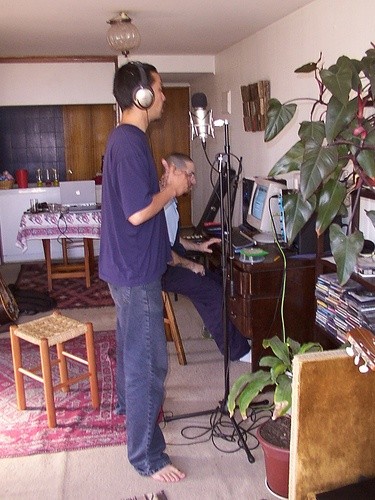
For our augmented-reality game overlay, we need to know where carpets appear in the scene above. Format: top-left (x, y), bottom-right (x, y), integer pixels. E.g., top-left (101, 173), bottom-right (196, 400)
top-left (16, 260), bottom-right (115, 309)
top-left (0, 330), bottom-right (165, 458)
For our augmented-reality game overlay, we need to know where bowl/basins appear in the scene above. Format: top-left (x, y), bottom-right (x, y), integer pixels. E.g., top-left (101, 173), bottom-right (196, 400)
top-left (48, 204), bottom-right (62, 213)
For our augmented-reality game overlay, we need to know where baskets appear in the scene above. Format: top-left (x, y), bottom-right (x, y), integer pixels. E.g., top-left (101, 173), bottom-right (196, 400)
top-left (0, 179), bottom-right (15, 189)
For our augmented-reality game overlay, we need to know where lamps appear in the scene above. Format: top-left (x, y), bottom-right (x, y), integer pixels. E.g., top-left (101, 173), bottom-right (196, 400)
top-left (105, 10), bottom-right (141, 58)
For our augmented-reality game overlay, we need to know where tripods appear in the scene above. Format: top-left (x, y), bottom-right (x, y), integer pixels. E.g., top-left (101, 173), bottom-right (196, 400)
top-left (164, 119), bottom-right (269, 463)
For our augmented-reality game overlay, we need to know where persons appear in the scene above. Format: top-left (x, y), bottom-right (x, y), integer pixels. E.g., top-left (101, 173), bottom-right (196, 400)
top-left (98, 63), bottom-right (191, 483)
top-left (161, 153), bottom-right (252, 363)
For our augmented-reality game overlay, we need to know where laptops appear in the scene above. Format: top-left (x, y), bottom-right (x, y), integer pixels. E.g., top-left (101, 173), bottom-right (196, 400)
top-left (59, 180), bottom-right (96, 207)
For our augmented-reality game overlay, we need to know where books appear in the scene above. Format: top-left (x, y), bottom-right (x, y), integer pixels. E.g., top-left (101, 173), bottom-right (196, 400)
top-left (315, 272), bottom-right (375, 344)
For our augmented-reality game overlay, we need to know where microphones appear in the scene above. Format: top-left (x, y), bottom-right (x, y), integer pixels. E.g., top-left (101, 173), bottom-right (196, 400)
top-left (191, 92), bottom-right (207, 148)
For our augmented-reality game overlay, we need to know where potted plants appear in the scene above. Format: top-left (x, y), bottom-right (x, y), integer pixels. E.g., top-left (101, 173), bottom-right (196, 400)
top-left (226, 336), bottom-right (351, 498)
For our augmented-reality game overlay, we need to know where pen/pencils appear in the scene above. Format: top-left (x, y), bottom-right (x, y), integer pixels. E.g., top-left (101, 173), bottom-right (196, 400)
top-left (274, 256), bottom-right (280, 261)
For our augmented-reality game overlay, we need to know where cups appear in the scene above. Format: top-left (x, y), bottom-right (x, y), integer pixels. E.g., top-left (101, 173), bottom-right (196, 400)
top-left (52, 168), bottom-right (59, 186)
top-left (30, 198), bottom-right (38, 214)
top-left (36, 168), bottom-right (43, 186)
top-left (45, 168), bottom-right (52, 187)
top-left (16, 169), bottom-right (28, 188)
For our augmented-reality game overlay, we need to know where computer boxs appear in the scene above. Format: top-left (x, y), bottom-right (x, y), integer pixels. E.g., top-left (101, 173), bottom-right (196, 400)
top-left (241, 177), bottom-right (258, 232)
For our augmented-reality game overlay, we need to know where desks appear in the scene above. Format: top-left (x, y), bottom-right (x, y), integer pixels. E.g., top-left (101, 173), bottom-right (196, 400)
top-left (15, 203), bottom-right (101, 290)
top-left (206, 227), bottom-right (343, 392)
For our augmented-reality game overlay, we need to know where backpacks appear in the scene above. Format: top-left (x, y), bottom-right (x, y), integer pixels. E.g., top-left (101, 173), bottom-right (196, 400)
top-left (9, 284), bottom-right (56, 315)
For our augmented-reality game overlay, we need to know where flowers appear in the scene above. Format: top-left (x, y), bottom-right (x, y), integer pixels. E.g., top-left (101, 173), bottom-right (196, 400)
top-left (0, 171), bottom-right (14, 181)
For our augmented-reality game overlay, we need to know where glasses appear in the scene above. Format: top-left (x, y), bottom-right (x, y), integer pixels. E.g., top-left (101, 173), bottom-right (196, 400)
top-left (187, 172), bottom-right (197, 178)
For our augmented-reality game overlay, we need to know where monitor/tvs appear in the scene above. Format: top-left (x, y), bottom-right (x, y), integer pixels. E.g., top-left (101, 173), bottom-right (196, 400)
top-left (246, 178), bottom-right (290, 244)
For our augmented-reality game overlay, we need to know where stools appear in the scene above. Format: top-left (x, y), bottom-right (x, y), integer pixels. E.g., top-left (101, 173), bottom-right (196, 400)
top-left (161, 291), bottom-right (187, 365)
top-left (9, 311), bottom-right (101, 428)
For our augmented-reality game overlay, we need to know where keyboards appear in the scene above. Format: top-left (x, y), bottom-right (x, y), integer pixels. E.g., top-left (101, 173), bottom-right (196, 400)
top-left (226, 231), bottom-right (257, 249)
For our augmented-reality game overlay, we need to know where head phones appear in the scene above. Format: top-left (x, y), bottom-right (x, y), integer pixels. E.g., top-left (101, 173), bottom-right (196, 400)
top-left (128, 61), bottom-right (155, 110)
